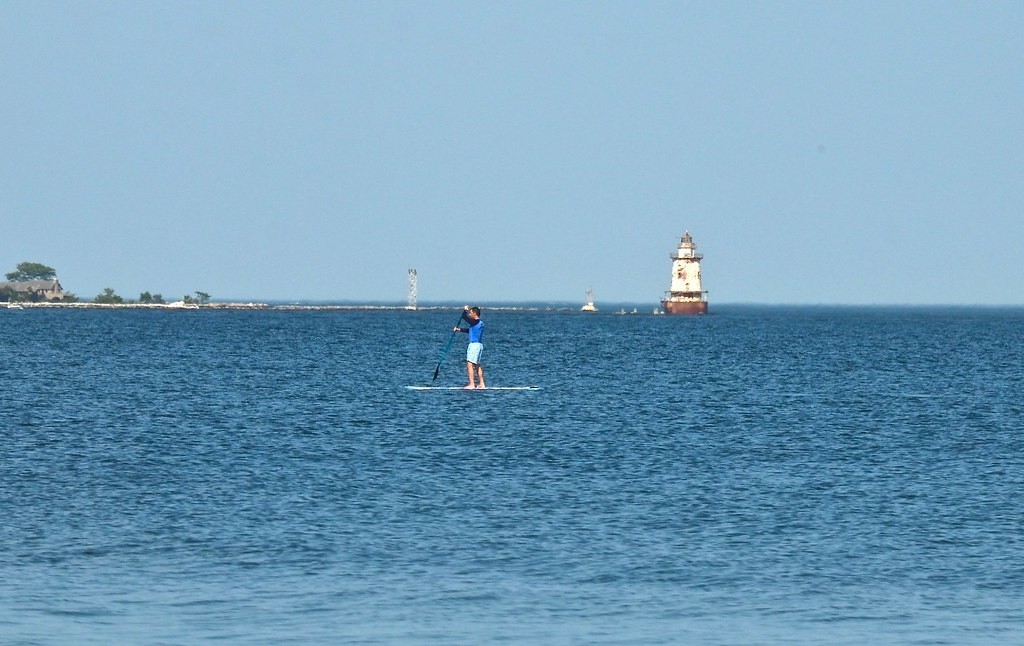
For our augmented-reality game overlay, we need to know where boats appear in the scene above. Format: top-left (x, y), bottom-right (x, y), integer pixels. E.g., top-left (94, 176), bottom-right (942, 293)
top-left (655, 231), bottom-right (710, 314)
top-left (580, 303), bottom-right (600, 312)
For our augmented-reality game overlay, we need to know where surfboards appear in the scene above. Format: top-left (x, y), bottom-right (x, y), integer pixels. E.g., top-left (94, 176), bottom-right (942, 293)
top-left (403, 385), bottom-right (541, 392)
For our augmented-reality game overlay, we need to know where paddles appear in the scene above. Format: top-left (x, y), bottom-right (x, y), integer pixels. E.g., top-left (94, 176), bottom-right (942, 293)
top-left (433, 309), bottom-right (465, 382)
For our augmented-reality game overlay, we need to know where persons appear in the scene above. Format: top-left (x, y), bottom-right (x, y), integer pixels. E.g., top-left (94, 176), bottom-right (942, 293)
top-left (454, 305), bottom-right (485, 388)
